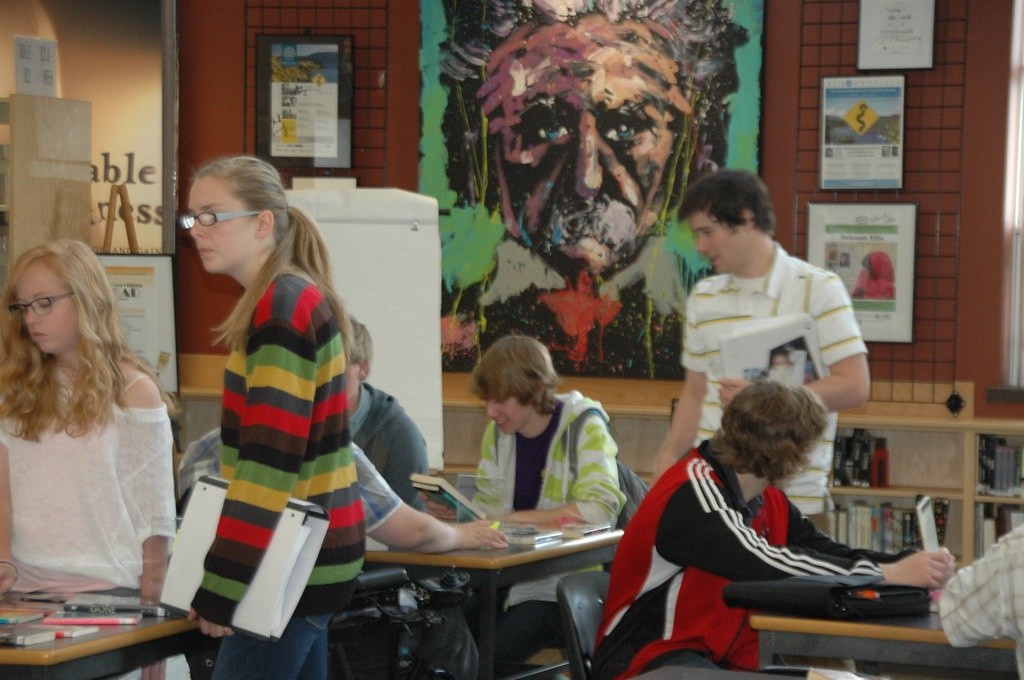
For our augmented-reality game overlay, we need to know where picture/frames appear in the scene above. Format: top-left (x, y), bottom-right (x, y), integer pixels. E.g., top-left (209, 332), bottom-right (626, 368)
top-left (806, 199), bottom-right (921, 344)
top-left (253, 32), bottom-right (355, 170)
top-left (816, 73), bottom-right (907, 194)
top-left (96, 252), bottom-right (181, 396)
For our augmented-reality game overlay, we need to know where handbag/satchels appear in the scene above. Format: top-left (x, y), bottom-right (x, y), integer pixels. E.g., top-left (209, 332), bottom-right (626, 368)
top-left (353, 568), bottom-right (424, 619)
top-left (723, 574), bottom-right (930, 621)
top-left (602, 458), bottom-right (648, 571)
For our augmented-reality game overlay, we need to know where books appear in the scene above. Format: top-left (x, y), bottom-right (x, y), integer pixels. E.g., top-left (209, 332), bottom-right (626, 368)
top-left (833, 428), bottom-right (887, 488)
top-left (826, 495), bottom-right (950, 555)
top-left (410, 473), bottom-right (487, 521)
top-left (0, 599), bottom-right (143, 646)
top-left (975, 502), bottom-right (1024, 560)
top-left (718, 314), bottom-right (825, 388)
top-left (978, 434), bottom-right (1024, 498)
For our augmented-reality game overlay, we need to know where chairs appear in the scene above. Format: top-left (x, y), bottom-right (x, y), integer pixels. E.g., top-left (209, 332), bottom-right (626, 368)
top-left (556, 570), bottom-right (610, 680)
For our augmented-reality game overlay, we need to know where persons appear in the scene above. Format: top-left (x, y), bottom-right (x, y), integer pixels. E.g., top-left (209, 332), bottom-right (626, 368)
top-left (590, 381), bottom-right (953, 680)
top-left (655, 171), bottom-right (872, 517)
top-left (470, 335), bottom-right (627, 680)
top-left (179, 157), bottom-right (366, 680)
top-left (939, 525), bottom-right (1024, 680)
top-left (177, 427), bottom-right (510, 553)
top-left (345, 313), bottom-right (430, 516)
top-left (0, 240), bottom-right (176, 680)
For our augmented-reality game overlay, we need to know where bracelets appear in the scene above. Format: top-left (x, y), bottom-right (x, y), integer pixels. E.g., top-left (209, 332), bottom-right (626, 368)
top-left (0, 561), bottom-right (17, 572)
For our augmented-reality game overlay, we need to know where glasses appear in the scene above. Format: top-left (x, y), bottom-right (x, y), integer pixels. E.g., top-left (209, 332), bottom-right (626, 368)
top-left (8, 291), bottom-right (76, 322)
top-left (178, 211), bottom-right (260, 229)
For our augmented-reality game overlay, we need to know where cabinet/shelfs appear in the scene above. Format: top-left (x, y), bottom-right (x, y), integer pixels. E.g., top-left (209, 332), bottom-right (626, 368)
top-left (0, 95), bottom-right (92, 300)
top-left (443, 400), bottom-right (1024, 568)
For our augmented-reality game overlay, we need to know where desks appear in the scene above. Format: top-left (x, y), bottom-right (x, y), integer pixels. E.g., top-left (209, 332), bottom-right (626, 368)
top-left (359, 525), bottom-right (627, 680)
top-left (748, 608), bottom-right (1018, 680)
top-left (0, 596), bottom-right (219, 680)
